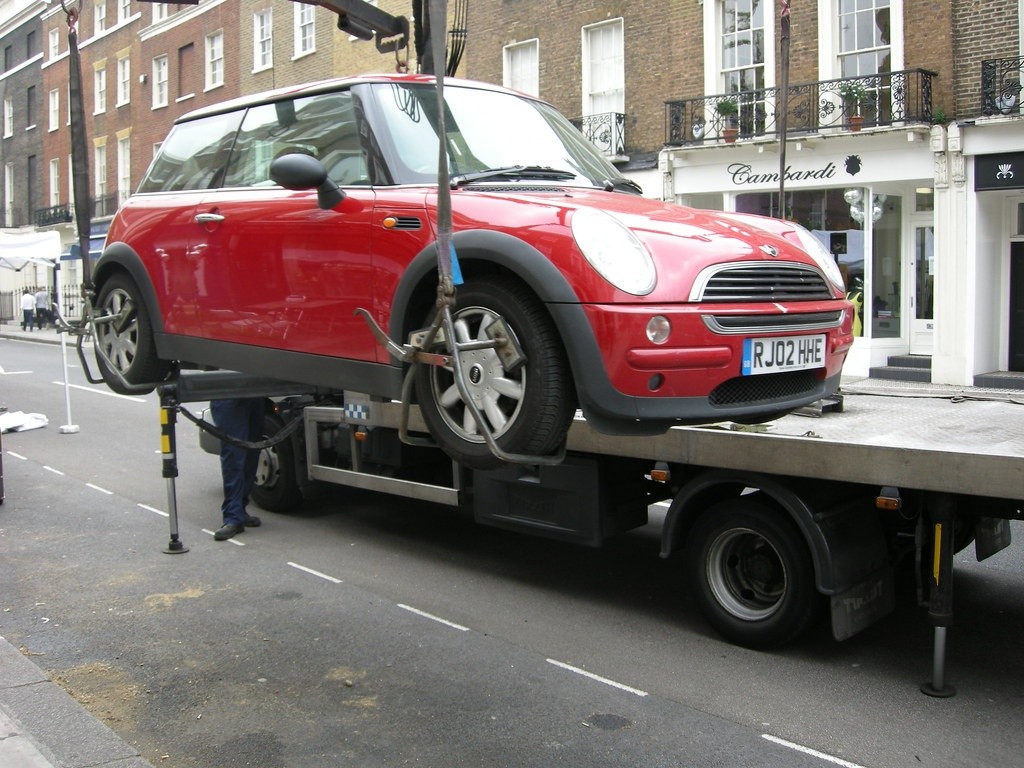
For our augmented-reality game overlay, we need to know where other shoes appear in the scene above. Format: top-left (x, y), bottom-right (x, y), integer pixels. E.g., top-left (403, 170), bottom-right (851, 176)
top-left (244, 515), bottom-right (260, 526)
top-left (215, 523), bottom-right (245, 540)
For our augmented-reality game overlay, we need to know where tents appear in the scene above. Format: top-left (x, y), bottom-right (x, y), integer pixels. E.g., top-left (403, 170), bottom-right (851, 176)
top-left (0, 228), bottom-right (61, 302)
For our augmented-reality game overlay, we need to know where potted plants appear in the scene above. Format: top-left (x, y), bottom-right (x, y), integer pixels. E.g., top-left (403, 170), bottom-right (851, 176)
top-left (714, 100), bottom-right (739, 143)
top-left (840, 82), bottom-right (872, 131)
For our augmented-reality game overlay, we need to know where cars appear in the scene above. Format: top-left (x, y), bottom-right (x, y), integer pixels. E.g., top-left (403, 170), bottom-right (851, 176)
top-left (81, 69), bottom-right (859, 466)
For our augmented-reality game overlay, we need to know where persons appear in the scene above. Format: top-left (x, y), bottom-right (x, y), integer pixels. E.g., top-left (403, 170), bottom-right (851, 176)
top-left (210, 395), bottom-right (270, 541)
top-left (35, 286), bottom-right (50, 329)
top-left (20, 290), bottom-right (37, 332)
top-left (845, 276), bottom-right (864, 337)
